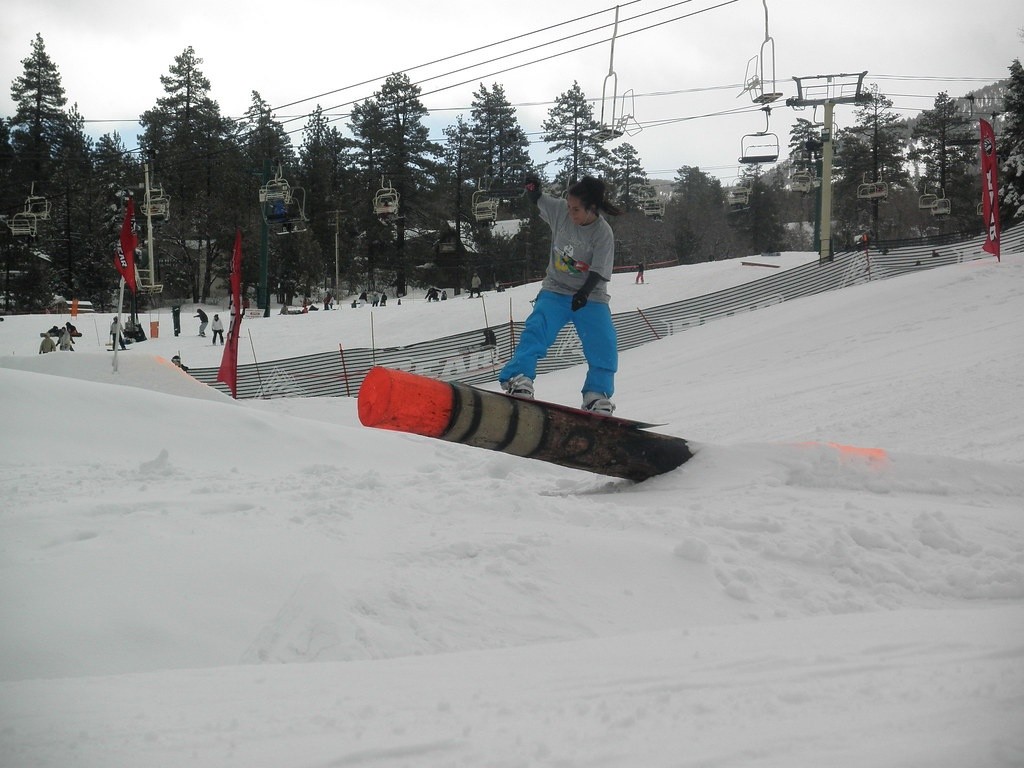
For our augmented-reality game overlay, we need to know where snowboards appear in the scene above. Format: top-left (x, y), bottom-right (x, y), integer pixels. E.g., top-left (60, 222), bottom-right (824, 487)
top-left (449, 380), bottom-right (669, 429)
top-left (107, 348), bottom-right (132, 352)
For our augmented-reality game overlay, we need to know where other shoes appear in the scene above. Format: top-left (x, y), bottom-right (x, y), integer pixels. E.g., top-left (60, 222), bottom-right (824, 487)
top-left (502, 377), bottom-right (532, 398)
top-left (580, 393), bottom-right (615, 415)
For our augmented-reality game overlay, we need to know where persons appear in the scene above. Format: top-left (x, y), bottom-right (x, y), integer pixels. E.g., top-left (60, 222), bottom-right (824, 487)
top-left (844, 228), bottom-right (875, 253)
top-left (636, 263), bottom-right (644, 282)
top-left (274, 199), bottom-right (298, 233)
top-left (378, 200), bottom-right (389, 221)
top-left (194, 309), bottom-right (208, 337)
top-left (171, 356), bottom-right (188, 372)
top-left (279, 289), bottom-right (387, 315)
top-left (211, 314), bottom-right (224, 345)
top-left (107, 316), bottom-right (130, 351)
top-left (469, 272), bottom-right (482, 298)
top-left (499, 175), bottom-right (622, 417)
top-left (39, 321), bottom-right (79, 354)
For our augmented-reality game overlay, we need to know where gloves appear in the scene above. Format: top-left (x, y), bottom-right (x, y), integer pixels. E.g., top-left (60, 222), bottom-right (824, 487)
top-left (571, 291), bottom-right (587, 312)
top-left (526, 178), bottom-right (541, 191)
